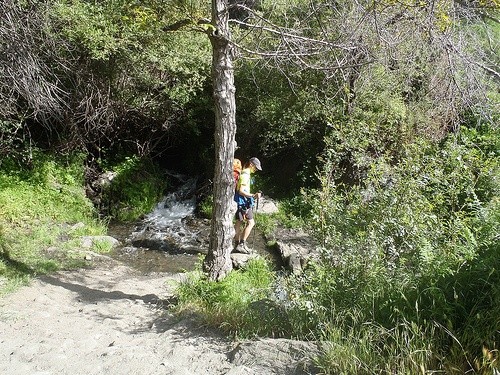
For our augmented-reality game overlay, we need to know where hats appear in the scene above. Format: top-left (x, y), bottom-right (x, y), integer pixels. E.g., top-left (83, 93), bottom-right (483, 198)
top-left (250, 157), bottom-right (262, 170)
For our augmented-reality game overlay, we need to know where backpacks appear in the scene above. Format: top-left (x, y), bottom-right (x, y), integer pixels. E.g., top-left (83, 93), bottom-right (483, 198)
top-left (233, 159), bottom-right (250, 188)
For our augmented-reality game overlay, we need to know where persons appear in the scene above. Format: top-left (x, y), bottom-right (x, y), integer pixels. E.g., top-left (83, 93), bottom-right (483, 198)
top-left (229, 156), bottom-right (262, 254)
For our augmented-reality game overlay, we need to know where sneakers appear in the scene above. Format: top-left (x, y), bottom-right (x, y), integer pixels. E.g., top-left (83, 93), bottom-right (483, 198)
top-left (232, 245), bottom-right (240, 253)
top-left (235, 243), bottom-right (250, 254)
